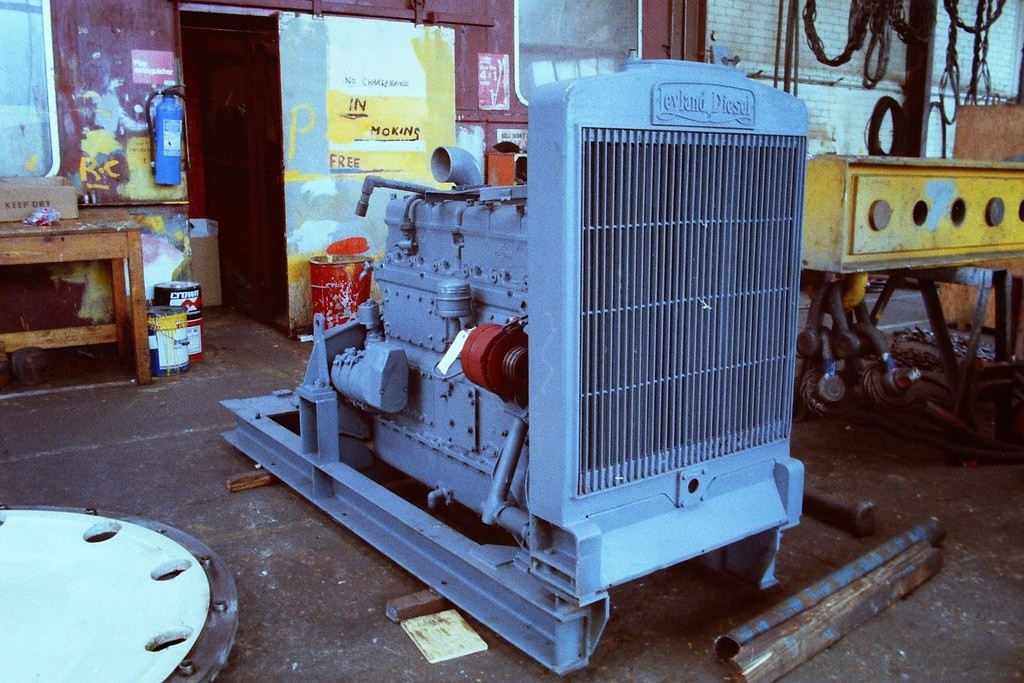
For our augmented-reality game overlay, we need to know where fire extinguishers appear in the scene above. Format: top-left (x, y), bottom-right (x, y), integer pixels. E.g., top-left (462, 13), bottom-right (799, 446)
top-left (146, 84), bottom-right (188, 185)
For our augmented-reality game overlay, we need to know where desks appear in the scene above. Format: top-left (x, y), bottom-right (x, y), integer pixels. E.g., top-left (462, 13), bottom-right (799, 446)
top-left (0, 211), bottom-right (153, 383)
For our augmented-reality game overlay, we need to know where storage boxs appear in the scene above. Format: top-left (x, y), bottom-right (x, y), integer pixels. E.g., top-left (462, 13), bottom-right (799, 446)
top-left (188, 217), bottom-right (222, 308)
top-left (0, 176), bottom-right (78, 223)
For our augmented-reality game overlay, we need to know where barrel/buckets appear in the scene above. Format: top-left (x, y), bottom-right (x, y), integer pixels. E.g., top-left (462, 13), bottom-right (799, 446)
top-left (311, 255), bottom-right (375, 328)
top-left (148, 305), bottom-right (191, 377)
top-left (155, 282), bottom-right (204, 361)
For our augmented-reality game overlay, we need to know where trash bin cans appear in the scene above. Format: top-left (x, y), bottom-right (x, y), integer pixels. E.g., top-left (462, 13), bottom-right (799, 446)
top-left (307, 253), bottom-right (374, 330)
